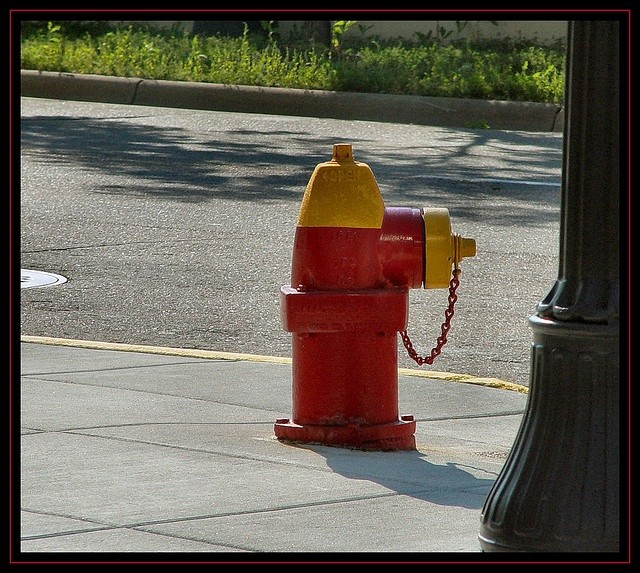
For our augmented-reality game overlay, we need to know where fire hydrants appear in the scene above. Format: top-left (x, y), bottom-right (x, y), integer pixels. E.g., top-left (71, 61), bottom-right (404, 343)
top-left (275, 145), bottom-right (475, 451)
